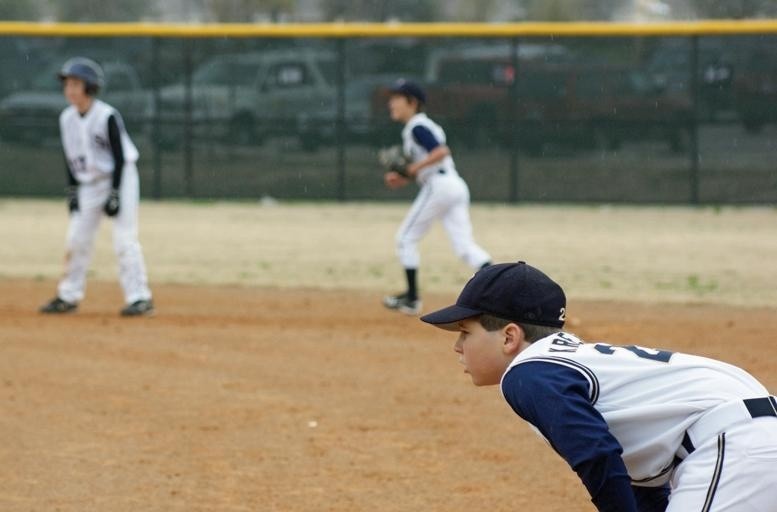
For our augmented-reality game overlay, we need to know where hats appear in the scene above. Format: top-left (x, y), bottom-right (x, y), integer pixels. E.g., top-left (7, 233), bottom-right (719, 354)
top-left (375, 78), bottom-right (424, 103)
top-left (419, 261), bottom-right (565, 330)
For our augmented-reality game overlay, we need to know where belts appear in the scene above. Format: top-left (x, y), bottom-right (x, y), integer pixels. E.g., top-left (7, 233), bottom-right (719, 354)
top-left (673, 397), bottom-right (777, 469)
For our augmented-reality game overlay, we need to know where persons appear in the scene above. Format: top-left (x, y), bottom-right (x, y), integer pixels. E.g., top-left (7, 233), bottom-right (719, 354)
top-left (420, 259), bottom-right (777, 511)
top-left (34, 56), bottom-right (155, 317)
top-left (376, 75), bottom-right (498, 318)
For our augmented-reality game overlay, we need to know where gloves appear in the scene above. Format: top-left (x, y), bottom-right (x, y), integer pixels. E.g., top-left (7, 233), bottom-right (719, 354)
top-left (66, 185), bottom-right (78, 211)
top-left (103, 188), bottom-right (118, 216)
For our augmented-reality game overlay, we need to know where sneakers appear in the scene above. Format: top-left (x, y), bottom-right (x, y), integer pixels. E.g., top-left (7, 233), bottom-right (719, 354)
top-left (120, 298), bottom-right (152, 315)
top-left (39, 298), bottom-right (75, 312)
top-left (382, 294), bottom-right (422, 316)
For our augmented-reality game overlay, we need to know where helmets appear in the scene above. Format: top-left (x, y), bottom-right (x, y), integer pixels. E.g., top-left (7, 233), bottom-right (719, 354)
top-left (54, 56), bottom-right (104, 88)
